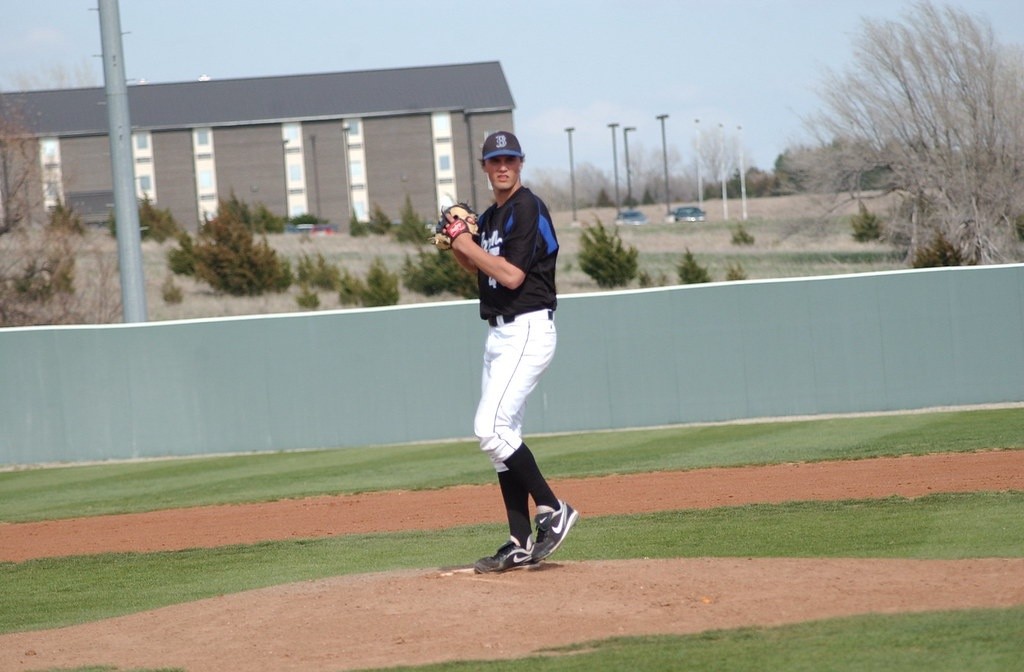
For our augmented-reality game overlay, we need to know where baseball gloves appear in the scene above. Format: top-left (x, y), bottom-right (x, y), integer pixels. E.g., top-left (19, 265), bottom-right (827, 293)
top-left (424, 190), bottom-right (482, 251)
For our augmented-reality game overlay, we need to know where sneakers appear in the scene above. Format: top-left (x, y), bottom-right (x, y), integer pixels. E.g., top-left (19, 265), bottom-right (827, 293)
top-left (475, 542), bottom-right (541, 573)
top-left (531, 499), bottom-right (578, 563)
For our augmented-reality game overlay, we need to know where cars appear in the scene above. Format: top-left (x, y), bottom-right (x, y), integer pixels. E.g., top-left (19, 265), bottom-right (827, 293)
top-left (614, 206), bottom-right (706, 226)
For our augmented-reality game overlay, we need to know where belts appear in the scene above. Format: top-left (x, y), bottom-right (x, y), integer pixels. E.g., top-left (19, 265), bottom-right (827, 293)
top-left (488, 309), bottom-right (553, 327)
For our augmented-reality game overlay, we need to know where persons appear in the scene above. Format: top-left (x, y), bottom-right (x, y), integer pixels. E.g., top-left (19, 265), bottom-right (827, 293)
top-left (429, 131), bottom-right (578, 573)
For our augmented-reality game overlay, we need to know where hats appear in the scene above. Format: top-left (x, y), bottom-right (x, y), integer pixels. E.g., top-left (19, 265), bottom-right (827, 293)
top-left (482, 132), bottom-right (524, 161)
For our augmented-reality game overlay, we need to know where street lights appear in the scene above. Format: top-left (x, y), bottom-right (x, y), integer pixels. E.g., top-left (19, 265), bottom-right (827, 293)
top-left (607, 122), bottom-right (636, 210)
top-left (694, 118), bottom-right (747, 221)
top-left (656, 115), bottom-right (672, 212)
top-left (564, 127), bottom-right (578, 221)
top-left (341, 122), bottom-right (351, 217)
